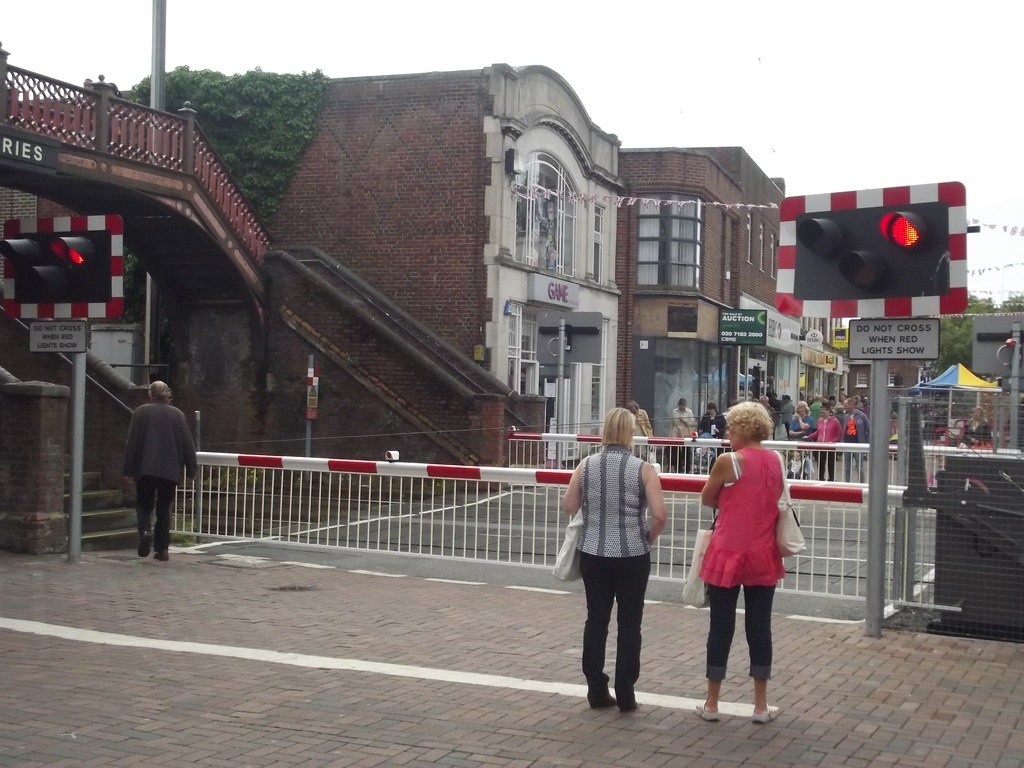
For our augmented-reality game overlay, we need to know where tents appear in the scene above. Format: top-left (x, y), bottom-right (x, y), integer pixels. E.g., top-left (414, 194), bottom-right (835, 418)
top-left (906, 362), bottom-right (999, 428)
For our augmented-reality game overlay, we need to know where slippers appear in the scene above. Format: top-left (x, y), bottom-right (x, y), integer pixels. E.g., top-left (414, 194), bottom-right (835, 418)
top-left (694, 700), bottom-right (720, 722)
top-left (751, 704), bottom-right (781, 724)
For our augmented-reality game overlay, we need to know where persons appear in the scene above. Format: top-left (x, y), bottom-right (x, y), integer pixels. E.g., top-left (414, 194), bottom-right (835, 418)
top-left (780, 394), bottom-right (794, 441)
top-left (671, 398), bottom-right (694, 474)
top-left (788, 401), bottom-right (813, 442)
top-left (769, 393), bottom-right (781, 410)
top-left (786, 451), bottom-right (803, 479)
top-left (693, 401), bottom-right (787, 723)
top-left (121, 381), bottom-right (197, 561)
top-left (802, 406), bottom-right (843, 481)
top-left (697, 402), bottom-right (726, 473)
top-left (807, 395), bottom-right (870, 462)
top-left (563, 401), bottom-right (666, 712)
top-left (962, 405), bottom-right (992, 450)
top-left (759, 395), bottom-right (778, 426)
top-left (840, 398), bottom-right (869, 484)
top-left (694, 447), bottom-right (708, 474)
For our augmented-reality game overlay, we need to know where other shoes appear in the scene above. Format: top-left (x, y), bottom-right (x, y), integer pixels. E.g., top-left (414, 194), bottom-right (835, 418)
top-left (137, 530), bottom-right (154, 557)
top-left (618, 703), bottom-right (639, 712)
top-left (153, 551), bottom-right (169, 561)
top-left (588, 697), bottom-right (617, 708)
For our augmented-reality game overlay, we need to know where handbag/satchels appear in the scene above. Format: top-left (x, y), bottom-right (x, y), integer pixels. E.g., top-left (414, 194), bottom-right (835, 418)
top-left (681, 506), bottom-right (719, 609)
top-left (772, 450), bottom-right (808, 557)
top-left (551, 506), bottom-right (584, 583)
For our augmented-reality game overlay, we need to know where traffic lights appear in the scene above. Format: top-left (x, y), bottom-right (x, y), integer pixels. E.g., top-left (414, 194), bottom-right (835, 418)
top-left (0, 229), bottom-right (112, 303)
top-left (792, 201), bottom-right (950, 300)
